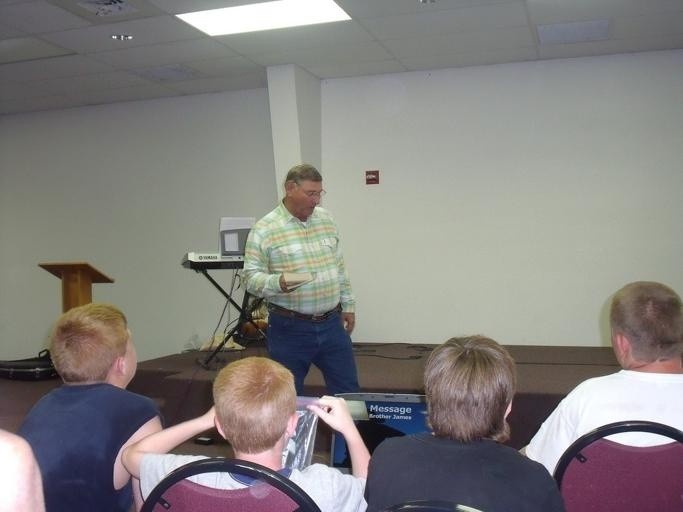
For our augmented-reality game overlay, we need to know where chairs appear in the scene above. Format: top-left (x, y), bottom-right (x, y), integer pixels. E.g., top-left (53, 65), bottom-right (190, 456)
top-left (383, 502), bottom-right (483, 512)
top-left (139, 458), bottom-right (323, 512)
top-left (553, 422), bottom-right (683, 512)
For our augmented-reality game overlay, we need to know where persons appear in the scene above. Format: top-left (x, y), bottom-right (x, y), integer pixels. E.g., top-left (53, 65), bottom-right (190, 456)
top-left (518, 281), bottom-right (683, 479)
top-left (16, 303), bottom-right (165, 512)
top-left (364, 336), bottom-right (566, 512)
top-left (121, 355), bottom-right (371, 512)
top-left (241, 164), bottom-right (361, 397)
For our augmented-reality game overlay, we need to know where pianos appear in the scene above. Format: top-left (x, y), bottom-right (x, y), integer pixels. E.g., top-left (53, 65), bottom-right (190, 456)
top-left (181, 252), bottom-right (244, 269)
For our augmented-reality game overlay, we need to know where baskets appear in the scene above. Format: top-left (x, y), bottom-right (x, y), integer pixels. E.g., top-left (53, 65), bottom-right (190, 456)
top-left (0, 360), bottom-right (58, 381)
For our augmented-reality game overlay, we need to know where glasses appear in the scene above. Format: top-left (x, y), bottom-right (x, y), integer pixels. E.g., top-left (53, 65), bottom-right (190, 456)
top-left (293, 181), bottom-right (327, 197)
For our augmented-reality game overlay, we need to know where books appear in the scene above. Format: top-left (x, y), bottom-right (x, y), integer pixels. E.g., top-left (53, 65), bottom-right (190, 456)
top-left (283, 272), bottom-right (317, 290)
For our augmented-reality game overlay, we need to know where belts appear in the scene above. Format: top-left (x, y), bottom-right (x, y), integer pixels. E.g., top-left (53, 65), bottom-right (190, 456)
top-left (269, 302), bottom-right (341, 322)
